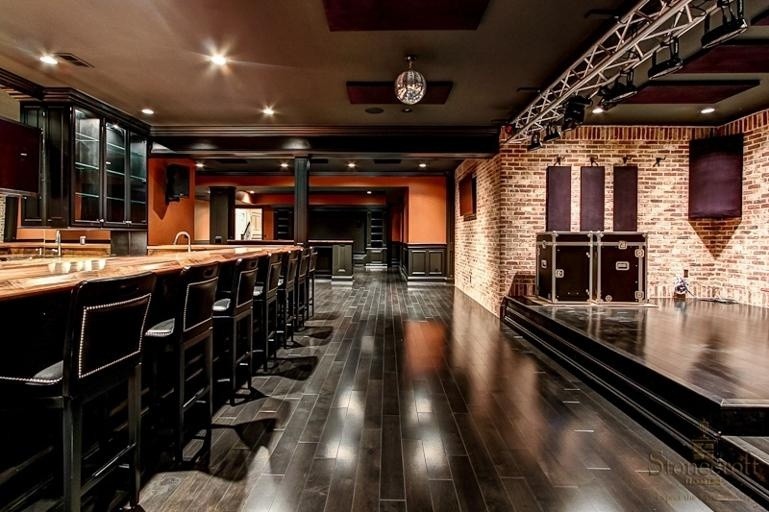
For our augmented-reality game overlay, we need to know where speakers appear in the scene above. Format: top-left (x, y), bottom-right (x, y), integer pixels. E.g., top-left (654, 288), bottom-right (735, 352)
top-left (166, 164), bottom-right (188, 201)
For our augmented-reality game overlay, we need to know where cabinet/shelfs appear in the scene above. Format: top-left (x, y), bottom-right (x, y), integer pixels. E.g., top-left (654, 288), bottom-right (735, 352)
top-left (104, 113), bottom-right (150, 230)
top-left (71, 99), bottom-right (104, 228)
top-left (19, 99), bottom-right (70, 229)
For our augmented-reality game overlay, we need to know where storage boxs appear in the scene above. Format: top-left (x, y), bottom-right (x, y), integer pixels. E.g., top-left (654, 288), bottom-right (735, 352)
top-left (533, 229), bottom-right (593, 307)
top-left (589, 229), bottom-right (650, 306)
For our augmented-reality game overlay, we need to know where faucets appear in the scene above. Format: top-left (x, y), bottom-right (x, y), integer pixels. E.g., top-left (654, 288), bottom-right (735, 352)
top-left (56, 231), bottom-right (61, 255)
top-left (173, 231), bottom-right (191, 252)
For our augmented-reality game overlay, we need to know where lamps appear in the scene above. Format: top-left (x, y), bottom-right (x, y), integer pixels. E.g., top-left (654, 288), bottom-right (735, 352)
top-left (543, 121), bottom-right (560, 143)
top-left (597, 69), bottom-right (638, 111)
top-left (647, 39), bottom-right (683, 81)
top-left (561, 95), bottom-right (594, 132)
top-left (700, 0), bottom-right (748, 50)
top-left (527, 132), bottom-right (543, 151)
top-left (394, 55), bottom-right (426, 106)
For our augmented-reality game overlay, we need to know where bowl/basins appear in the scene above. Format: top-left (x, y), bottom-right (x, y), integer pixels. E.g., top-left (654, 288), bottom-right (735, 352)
top-left (48, 262), bottom-right (70, 274)
top-left (92, 259), bottom-right (106, 270)
top-left (70, 260), bottom-right (83, 272)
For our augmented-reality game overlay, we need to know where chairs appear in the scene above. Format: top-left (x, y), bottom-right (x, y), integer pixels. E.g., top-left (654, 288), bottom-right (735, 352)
top-left (0, 270), bottom-right (158, 511)
top-left (224, 251), bottom-right (284, 371)
top-left (280, 247), bottom-right (311, 326)
top-left (212, 255), bottom-right (260, 406)
top-left (142, 260), bottom-right (222, 465)
top-left (256, 249), bottom-right (300, 349)
top-left (306, 246), bottom-right (318, 320)
